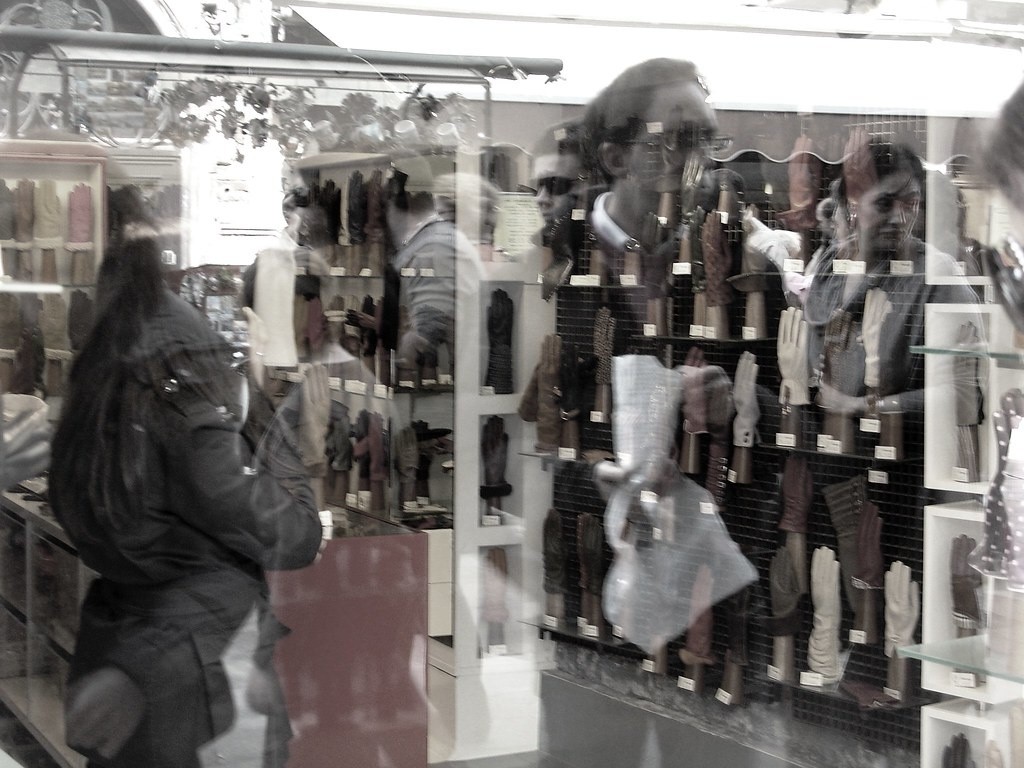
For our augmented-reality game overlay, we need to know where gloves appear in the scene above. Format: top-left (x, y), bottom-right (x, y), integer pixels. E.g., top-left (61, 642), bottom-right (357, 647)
top-left (953, 322), bottom-right (985, 427)
top-left (520, 128), bottom-right (930, 693)
top-left (948, 534), bottom-right (982, 622)
top-left (0, 175), bottom-right (100, 394)
top-left (241, 168), bottom-right (515, 512)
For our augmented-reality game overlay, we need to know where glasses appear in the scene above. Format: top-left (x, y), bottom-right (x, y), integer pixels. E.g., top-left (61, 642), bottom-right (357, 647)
top-left (536, 176), bottom-right (596, 195)
top-left (622, 125), bottom-right (719, 152)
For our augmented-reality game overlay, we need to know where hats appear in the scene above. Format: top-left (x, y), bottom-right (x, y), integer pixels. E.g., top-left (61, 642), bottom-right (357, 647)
top-left (376, 146), bottom-right (439, 192)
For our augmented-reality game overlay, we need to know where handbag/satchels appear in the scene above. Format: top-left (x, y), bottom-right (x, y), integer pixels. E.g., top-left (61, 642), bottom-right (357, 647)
top-left (599, 461), bottom-right (759, 656)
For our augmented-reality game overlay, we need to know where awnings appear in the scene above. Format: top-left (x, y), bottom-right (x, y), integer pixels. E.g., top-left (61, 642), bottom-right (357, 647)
top-left (290, 6), bottom-right (1024, 172)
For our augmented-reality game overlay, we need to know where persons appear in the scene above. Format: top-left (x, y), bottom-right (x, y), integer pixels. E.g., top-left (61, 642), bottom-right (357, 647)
top-left (51, 216), bottom-right (331, 768)
top-left (243, 57), bottom-right (1024, 768)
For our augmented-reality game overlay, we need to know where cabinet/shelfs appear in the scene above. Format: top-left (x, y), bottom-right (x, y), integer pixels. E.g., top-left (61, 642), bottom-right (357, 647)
top-left (0, 135), bottom-right (1024, 768)
